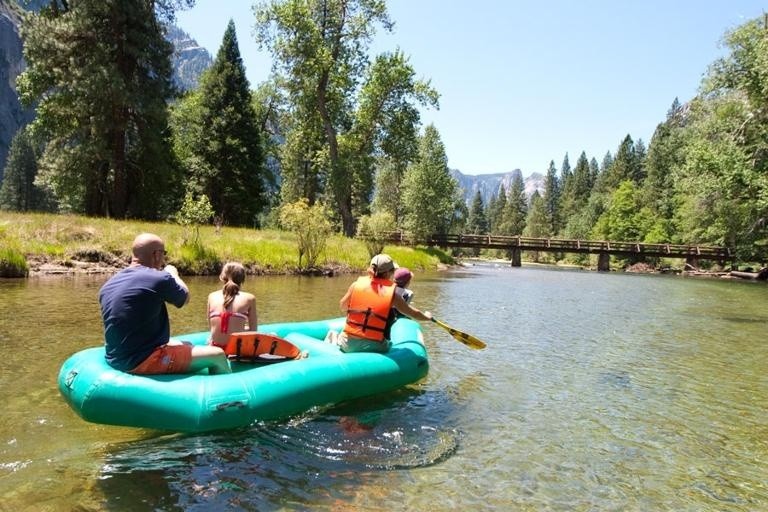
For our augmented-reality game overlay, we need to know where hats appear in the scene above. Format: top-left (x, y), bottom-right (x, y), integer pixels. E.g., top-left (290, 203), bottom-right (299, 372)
top-left (371, 255), bottom-right (398, 273)
top-left (393, 267), bottom-right (412, 284)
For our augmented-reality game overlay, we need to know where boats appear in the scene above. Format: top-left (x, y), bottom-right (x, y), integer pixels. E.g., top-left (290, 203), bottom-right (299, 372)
top-left (57, 316), bottom-right (427, 432)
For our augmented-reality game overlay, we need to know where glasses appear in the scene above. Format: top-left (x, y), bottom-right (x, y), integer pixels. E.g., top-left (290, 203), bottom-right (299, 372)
top-left (154, 249), bottom-right (167, 256)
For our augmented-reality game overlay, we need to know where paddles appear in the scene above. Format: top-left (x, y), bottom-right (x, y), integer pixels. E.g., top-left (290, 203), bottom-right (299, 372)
top-left (410, 307), bottom-right (487, 350)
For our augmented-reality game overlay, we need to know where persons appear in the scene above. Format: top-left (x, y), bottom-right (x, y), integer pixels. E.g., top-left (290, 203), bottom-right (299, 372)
top-left (206, 261), bottom-right (260, 359)
top-left (98, 233), bottom-right (233, 376)
top-left (336, 252), bottom-right (433, 355)
top-left (389, 267), bottom-right (416, 326)
